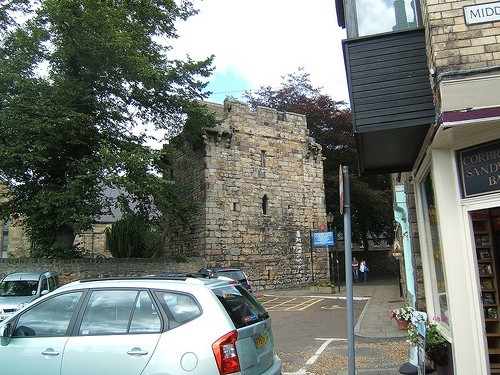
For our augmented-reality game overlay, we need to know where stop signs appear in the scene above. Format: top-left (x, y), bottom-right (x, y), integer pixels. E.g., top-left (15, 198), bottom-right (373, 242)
top-left (338, 164), bottom-right (344, 215)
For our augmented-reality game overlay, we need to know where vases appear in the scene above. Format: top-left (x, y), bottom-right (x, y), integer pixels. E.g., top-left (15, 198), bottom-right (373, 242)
top-left (395, 320), bottom-right (409, 330)
top-left (425, 348), bottom-right (447, 367)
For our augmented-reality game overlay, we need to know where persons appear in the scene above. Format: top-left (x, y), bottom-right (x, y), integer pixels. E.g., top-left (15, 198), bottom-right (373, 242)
top-left (352, 257), bottom-right (360, 283)
top-left (359, 260), bottom-right (370, 284)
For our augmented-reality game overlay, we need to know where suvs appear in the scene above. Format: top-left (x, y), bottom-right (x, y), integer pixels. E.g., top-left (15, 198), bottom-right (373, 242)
top-left (197, 266), bottom-right (252, 295)
top-left (0, 270), bottom-right (60, 324)
top-left (0, 277), bottom-right (283, 375)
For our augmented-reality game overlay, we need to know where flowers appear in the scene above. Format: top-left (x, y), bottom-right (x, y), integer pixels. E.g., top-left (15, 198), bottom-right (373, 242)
top-left (389, 307), bottom-right (415, 323)
top-left (406, 313), bottom-right (448, 352)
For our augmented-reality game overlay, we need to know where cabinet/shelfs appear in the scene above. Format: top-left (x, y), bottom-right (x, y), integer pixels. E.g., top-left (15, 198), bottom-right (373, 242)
top-left (470, 211), bottom-right (500, 375)
